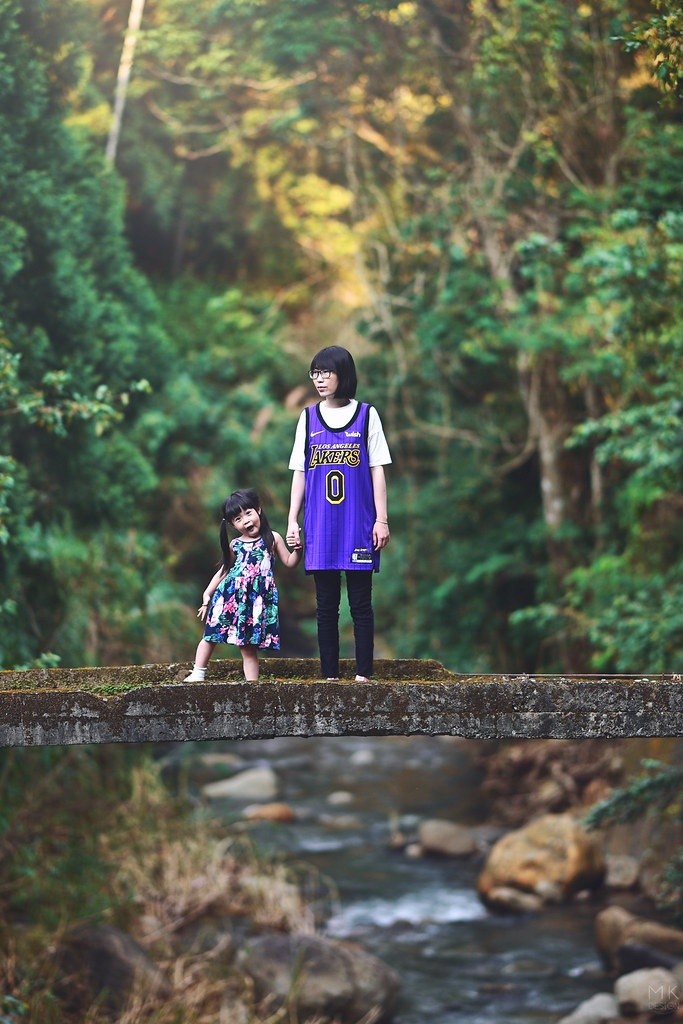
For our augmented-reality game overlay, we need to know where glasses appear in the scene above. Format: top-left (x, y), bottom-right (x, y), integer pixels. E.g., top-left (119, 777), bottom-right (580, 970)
top-left (308, 369), bottom-right (334, 379)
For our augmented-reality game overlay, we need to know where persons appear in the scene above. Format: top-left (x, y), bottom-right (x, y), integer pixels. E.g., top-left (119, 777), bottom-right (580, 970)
top-left (284, 346), bottom-right (393, 681)
top-left (181, 488), bottom-right (303, 683)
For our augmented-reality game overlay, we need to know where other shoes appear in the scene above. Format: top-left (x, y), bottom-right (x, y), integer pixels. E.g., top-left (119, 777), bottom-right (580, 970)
top-left (183, 674), bottom-right (205, 682)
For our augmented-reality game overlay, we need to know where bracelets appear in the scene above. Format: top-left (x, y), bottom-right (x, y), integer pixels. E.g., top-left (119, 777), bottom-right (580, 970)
top-left (375, 519), bottom-right (389, 525)
